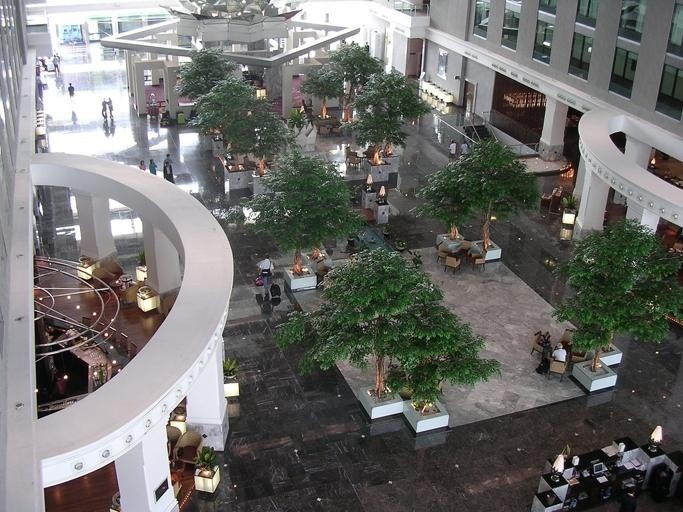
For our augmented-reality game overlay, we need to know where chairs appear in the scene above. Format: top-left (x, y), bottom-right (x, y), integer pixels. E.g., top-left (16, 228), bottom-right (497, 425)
top-left (315, 259), bottom-right (334, 292)
top-left (419, 90), bottom-right (452, 114)
top-left (532, 330), bottom-right (587, 382)
top-left (177, 113), bottom-right (184, 124)
top-left (149, 107), bottom-right (159, 121)
top-left (421, 80), bottom-right (454, 106)
top-left (226, 156), bottom-right (259, 171)
top-left (92, 257), bottom-right (144, 304)
top-left (345, 145), bottom-right (373, 170)
top-left (319, 124), bottom-right (345, 138)
top-left (437, 241), bottom-right (487, 275)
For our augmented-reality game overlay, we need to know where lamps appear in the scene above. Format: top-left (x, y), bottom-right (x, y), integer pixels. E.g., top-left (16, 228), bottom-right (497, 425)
top-left (550, 454), bottom-right (564, 482)
top-left (648, 425), bottom-right (663, 452)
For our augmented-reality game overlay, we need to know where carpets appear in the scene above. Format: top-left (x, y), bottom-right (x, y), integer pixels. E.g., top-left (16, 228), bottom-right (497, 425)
top-left (293, 247), bottom-right (587, 428)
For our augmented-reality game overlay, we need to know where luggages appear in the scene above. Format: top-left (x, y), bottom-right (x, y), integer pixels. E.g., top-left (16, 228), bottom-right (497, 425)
top-left (271, 284), bottom-right (281, 298)
top-left (256, 276), bottom-right (264, 287)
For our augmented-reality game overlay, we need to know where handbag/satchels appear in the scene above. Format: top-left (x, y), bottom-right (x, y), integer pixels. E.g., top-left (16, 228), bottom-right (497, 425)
top-left (262, 269), bottom-right (271, 277)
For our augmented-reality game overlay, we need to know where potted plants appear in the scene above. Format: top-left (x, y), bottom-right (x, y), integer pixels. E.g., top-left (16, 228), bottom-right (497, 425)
top-left (559, 194), bottom-right (579, 224)
top-left (192, 445), bottom-right (221, 493)
top-left (222, 356), bottom-right (240, 397)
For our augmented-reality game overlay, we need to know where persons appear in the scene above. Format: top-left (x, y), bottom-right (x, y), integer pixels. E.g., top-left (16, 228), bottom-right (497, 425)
top-left (72, 110), bottom-right (77, 125)
top-left (101, 98), bottom-right (114, 118)
top-left (619, 486), bottom-right (641, 512)
top-left (258, 255), bottom-right (274, 296)
top-left (448, 139), bottom-right (457, 163)
top-left (165, 98), bottom-right (169, 117)
top-left (460, 140), bottom-right (468, 158)
top-left (40, 53), bottom-right (60, 72)
top-left (536, 331), bottom-right (567, 375)
top-left (259, 298), bottom-right (274, 323)
top-left (68, 83), bottom-right (75, 97)
top-left (103, 119), bottom-right (115, 137)
top-left (139, 154), bottom-right (175, 184)
